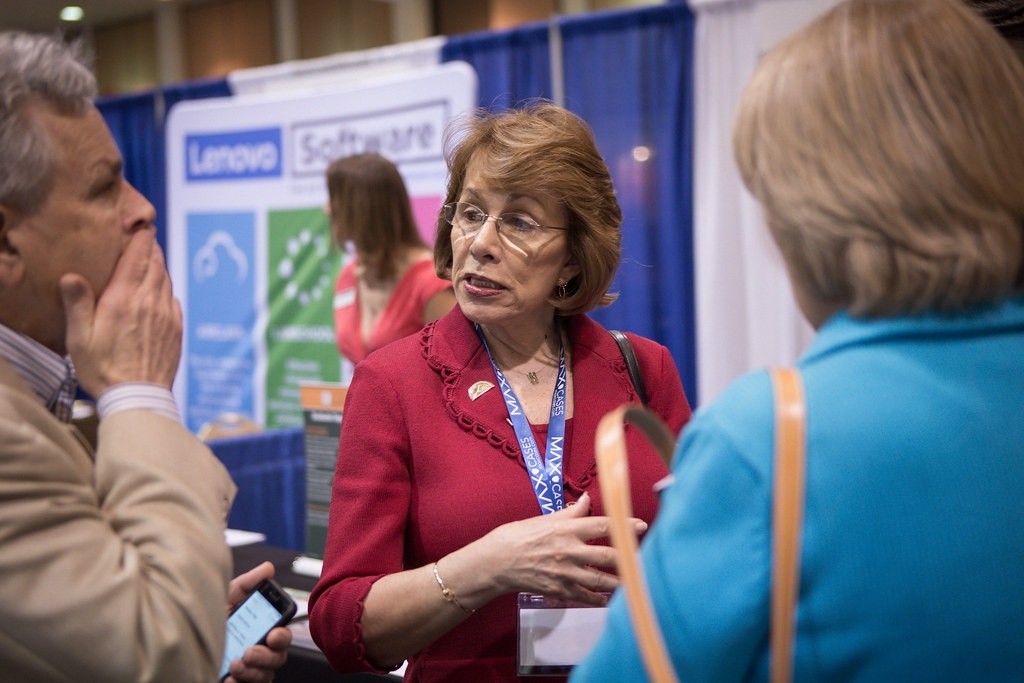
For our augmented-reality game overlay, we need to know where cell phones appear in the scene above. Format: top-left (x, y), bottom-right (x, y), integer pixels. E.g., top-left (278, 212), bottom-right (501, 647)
top-left (218, 577), bottom-right (298, 682)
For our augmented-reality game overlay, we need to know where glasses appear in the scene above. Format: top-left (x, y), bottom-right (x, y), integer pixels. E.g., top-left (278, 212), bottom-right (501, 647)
top-left (444, 202), bottom-right (568, 238)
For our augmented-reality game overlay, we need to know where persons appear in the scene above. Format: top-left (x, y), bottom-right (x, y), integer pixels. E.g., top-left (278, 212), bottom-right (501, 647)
top-left (0, 26), bottom-right (291, 683)
top-left (307, 108), bottom-right (696, 682)
top-left (322, 152), bottom-right (458, 368)
top-left (572, 0), bottom-right (1024, 682)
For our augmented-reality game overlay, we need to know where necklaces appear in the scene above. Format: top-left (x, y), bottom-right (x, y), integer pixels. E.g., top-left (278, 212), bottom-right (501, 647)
top-left (490, 338), bottom-right (560, 384)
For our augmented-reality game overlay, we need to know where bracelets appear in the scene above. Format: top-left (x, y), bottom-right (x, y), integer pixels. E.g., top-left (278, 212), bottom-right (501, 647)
top-left (433, 561), bottom-right (475, 616)
